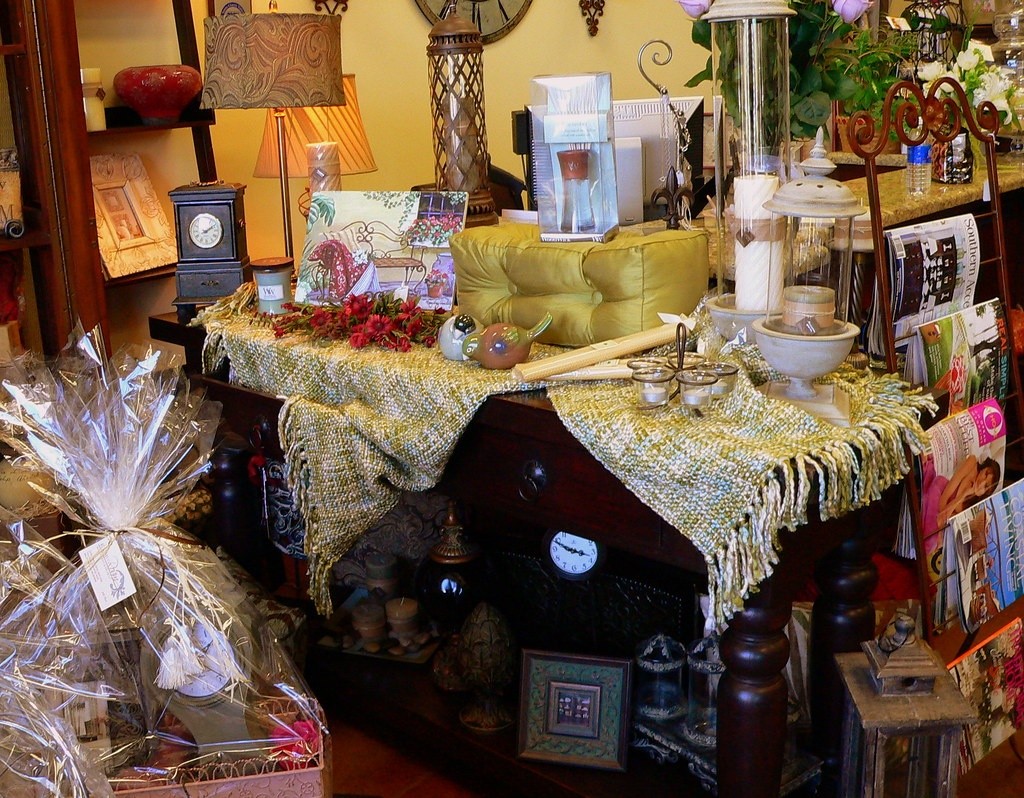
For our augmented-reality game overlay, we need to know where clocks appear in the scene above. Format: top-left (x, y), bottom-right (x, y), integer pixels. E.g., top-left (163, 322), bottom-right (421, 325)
top-left (548, 530), bottom-right (599, 575)
top-left (189, 213), bottom-right (224, 249)
top-left (416, 0), bottom-right (535, 45)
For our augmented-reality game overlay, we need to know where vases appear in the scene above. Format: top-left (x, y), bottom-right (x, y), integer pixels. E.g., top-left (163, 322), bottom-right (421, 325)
top-left (726, 145), bottom-right (802, 248)
top-left (928, 126), bottom-right (974, 183)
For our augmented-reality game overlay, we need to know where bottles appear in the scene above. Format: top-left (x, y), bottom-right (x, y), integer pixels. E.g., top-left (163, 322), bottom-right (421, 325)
top-left (557, 150), bottom-right (594, 232)
top-left (907, 118), bottom-right (933, 198)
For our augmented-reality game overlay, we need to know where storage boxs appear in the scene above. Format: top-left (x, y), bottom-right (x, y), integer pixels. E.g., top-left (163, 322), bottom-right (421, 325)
top-left (101, 696), bottom-right (334, 798)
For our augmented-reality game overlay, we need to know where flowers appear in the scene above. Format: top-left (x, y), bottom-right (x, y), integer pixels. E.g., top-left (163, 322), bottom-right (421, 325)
top-left (918, 38), bottom-right (1023, 131)
top-left (678, 0), bottom-right (900, 164)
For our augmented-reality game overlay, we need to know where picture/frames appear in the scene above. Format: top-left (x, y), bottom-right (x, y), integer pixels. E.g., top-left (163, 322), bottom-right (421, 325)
top-left (90, 153), bottom-right (178, 281)
top-left (520, 647), bottom-right (634, 774)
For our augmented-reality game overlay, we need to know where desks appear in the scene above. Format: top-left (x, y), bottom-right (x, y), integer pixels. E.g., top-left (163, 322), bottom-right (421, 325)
top-left (148, 302), bottom-right (929, 798)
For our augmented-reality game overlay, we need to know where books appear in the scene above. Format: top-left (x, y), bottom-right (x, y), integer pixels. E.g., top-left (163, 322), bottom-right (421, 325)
top-left (879, 210), bottom-right (1024, 630)
top-left (936, 617), bottom-right (1024, 777)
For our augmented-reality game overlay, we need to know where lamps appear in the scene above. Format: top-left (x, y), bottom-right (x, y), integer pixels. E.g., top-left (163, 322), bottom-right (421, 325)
top-left (251, 74), bottom-right (379, 218)
top-left (197, 14), bottom-right (345, 256)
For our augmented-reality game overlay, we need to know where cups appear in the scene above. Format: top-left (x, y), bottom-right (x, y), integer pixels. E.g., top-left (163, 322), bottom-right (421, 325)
top-left (250, 257), bottom-right (293, 316)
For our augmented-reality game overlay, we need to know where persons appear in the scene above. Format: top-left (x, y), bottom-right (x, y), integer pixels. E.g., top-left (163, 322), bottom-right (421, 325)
top-left (922, 448), bottom-right (1002, 557)
